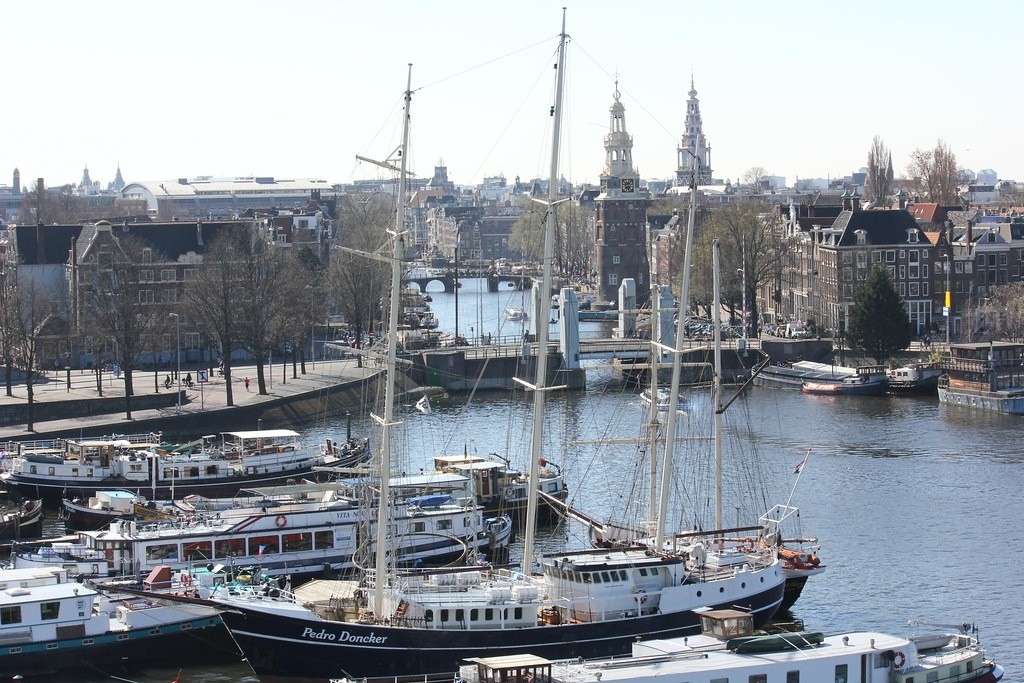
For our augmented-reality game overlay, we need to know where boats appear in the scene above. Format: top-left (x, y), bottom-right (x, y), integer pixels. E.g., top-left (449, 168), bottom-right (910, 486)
top-left (0, 413), bottom-right (371, 512)
top-left (389, 287), bottom-right (433, 325)
top-left (60, 445), bottom-right (569, 536)
top-left (640, 386), bottom-right (691, 412)
top-left (453, 281), bottom-right (462, 288)
top-left (792, 359), bottom-right (942, 395)
top-left (505, 308), bottom-right (529, 321)
top-left (750, 357), bottom-right (890, 396)
top-left (937, 339), bottom-right (1024, 414)
top-left (381, 605), bottom-right (1005, 682)
top-left (8, 494), bottom-right (512, 594)
top-left (0, 565), bottom-right (292, 676)
top-left (801, 382), bottom-right (840, 398)
top-left (508, 283), bottom-right (514, 287)
top-left (419, 312), bottom-right (440, 329)
top-left (1, 494), bottom-right (43, 551)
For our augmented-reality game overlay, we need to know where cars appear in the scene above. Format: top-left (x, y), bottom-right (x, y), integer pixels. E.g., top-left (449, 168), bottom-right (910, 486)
top-left (675, 314), bottom-right (812, 341)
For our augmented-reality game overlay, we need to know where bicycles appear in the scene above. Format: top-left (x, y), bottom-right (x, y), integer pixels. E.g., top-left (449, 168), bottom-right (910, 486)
top-left (158, 382), bottom-right (175, 392)
top-left (180, 380), bottom-right (196, 390)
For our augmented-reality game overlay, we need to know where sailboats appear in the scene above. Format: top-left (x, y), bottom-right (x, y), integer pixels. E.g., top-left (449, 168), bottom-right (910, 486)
top-left (206, 10), bottom-right (826, 683)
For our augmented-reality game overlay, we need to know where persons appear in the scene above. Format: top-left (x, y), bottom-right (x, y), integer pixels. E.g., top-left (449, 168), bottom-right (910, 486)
top-left (165, 374), bottom-right (170, 385)
top-left (244, 377), bottom-right (250, 392)
top-left (185, 373), bottom-right (192, 387)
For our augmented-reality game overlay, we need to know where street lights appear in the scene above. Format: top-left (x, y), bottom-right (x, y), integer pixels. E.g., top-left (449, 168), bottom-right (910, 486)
top-left (943, 254), bottom-right (949, 344)
top-left (169, 313), bottom-right (182, 413)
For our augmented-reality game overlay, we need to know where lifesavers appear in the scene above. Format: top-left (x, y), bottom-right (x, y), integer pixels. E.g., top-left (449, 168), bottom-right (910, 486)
top-left (742, 537), bottom-right (754, 551)
top-left (276, 515), bottom-right (287, 527)
top-left (505, 488), bottom-right (515, 497)
top-left (893, 651), bottom-right (906, 668)
top-left (181, 573), bottom-right (191, 587)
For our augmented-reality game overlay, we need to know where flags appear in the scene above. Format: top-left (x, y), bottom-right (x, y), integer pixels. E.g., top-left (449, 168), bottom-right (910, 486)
top-left (793, 461), bottom-right (804, 474)
